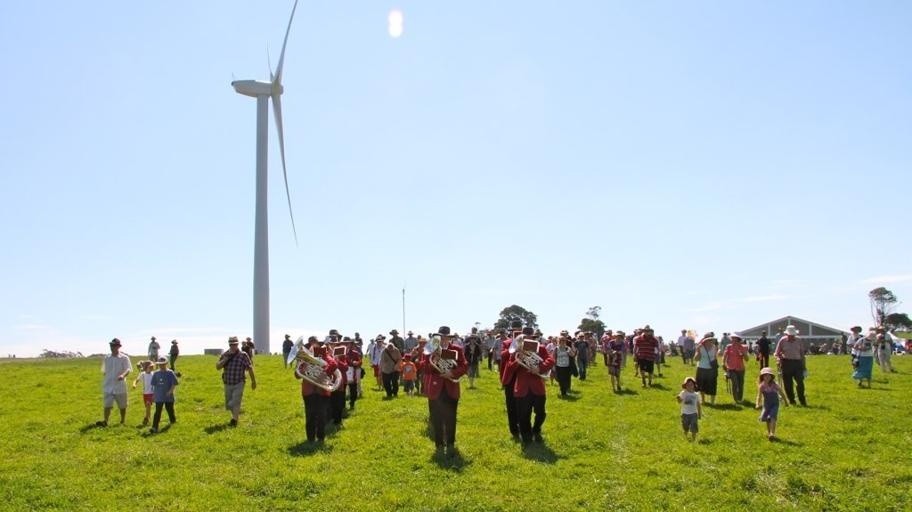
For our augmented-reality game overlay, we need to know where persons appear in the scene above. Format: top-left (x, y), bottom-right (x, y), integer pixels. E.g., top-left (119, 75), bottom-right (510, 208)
top-left (678, 329), bottom-right (771, 406)
top-left (847, 326), bottom-right (893, 388)
top-left (756, 368), bottom-right (788, 439)
top-left (808, 332), bottom-right (847, 355)
top-left (677, 376), bottom-right (701, 439)
top-left (132, 360), bottom-right (156, 424)
top-left (149, 356), bottom-right (179, 434)
top-left (96, 337), bottom-right (131, 424)
top-left (465, 328), bottom-right (556, 442)
top-left (246, 337), bottom-right (255, 355)
top-left (774, 326), bottom-right (807, 406)
top-left (282, 321), bottom-right (362, 441)
top-left (545, 326), bottom-right (666, 398)
top-left (366, 325), bottom-right (468, 454)
top-left (217, 335), bottom-right (256, 426)
top-left (241, 341), bottom-right (251, 360)
top-left (148, 336), bottom-right (160, 362)
top-left (168, 340), bottom-right (179, 369)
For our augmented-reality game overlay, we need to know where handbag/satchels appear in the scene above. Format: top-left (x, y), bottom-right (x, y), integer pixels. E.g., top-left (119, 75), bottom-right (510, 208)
top-left (852, 358), bottom-right (859, 366)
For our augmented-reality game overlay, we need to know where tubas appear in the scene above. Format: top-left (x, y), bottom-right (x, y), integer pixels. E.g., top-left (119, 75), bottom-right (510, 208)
top-left (287, 337), bottom-right (342, 392)
top-left (423, 336), bottom-right (465, 384)
top-left (510, 334), bottom-right (551, 379)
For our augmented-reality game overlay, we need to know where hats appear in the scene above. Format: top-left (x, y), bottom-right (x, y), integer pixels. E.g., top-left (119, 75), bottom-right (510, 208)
top-left (560, 330), bottom-right (569, 334)
top-left (304, 329), bottom-right (360, 348)
top-left (727, 331), bottom-right (743, 344)
top-left (699, 332), bottom-right (717, 346)
top-left (155, 357), bottom-right (168, 364)
top-left (682, 376), bottom-right (698, 389)
top-left (783, 325), bottom-right (798, 336)
top-left (150, 337), bottom-right (156, 340)
top-left (109, 336), bottom-right (122, 348)
top-left (171, 339), bottom-right (177, 344)
top-left (614, 331), bottom-right (625, 337)
top-left (507, 319), bottom-right (543, 338)
top-left (760, 367), bottom-right (775, 377)
top-left (433, 326), bottom-right (455, 337)
top-left (229, 336), bottom-right (240, 344)
top-left (376, 329), bottom-right (427, 359)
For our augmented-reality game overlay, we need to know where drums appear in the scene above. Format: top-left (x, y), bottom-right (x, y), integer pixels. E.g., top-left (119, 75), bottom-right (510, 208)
top-left (346, 367), bottom-right (354, 383)
